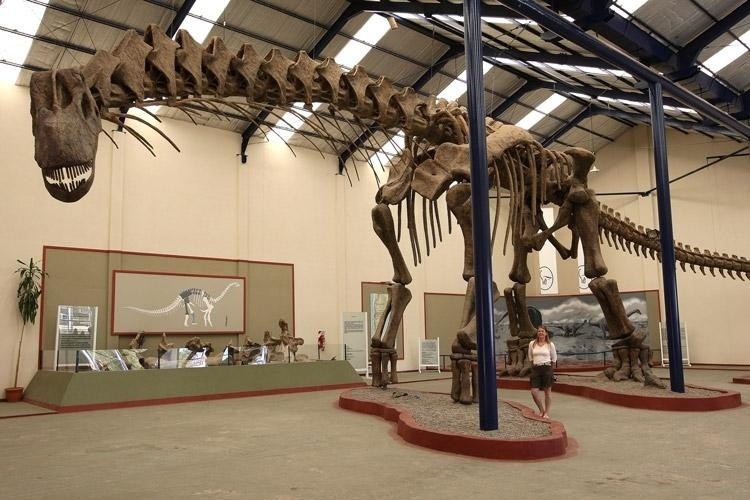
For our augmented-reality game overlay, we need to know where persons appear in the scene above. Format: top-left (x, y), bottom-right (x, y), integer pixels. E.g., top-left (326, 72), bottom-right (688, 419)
top-left (526, 325), bottom-right (556, 420)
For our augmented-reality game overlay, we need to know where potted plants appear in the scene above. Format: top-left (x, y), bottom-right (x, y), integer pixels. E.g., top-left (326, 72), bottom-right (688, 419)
top-left (3, 258), bottom-right (51, 401)
